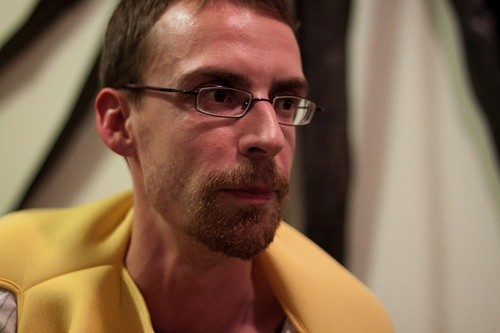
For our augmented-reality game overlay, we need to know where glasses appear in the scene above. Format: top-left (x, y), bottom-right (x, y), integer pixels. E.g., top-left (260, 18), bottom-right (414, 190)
top-left (120, 84), bottom-right (316, 126)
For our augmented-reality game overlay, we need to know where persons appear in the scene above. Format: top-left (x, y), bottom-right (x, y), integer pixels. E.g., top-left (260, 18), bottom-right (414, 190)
top-left (1, 0), bottom-right (396, 333)
